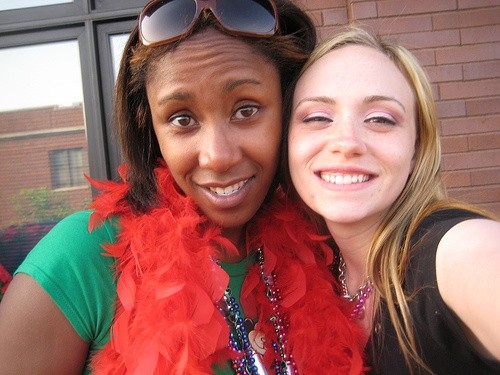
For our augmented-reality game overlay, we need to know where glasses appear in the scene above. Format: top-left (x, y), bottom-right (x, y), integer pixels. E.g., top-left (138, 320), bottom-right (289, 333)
top-left (138, 0), bottom-right (279, 46)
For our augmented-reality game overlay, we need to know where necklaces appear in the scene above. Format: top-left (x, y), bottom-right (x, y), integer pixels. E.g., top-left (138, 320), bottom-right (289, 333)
top-left (82, 158), bottom-right (372, 375)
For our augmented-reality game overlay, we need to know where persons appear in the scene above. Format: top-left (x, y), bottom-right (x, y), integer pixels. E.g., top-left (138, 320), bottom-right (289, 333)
top-left (0, 0), bottom-right (361, 375)
top-left (287, 23), bottom-right (500, 375)
